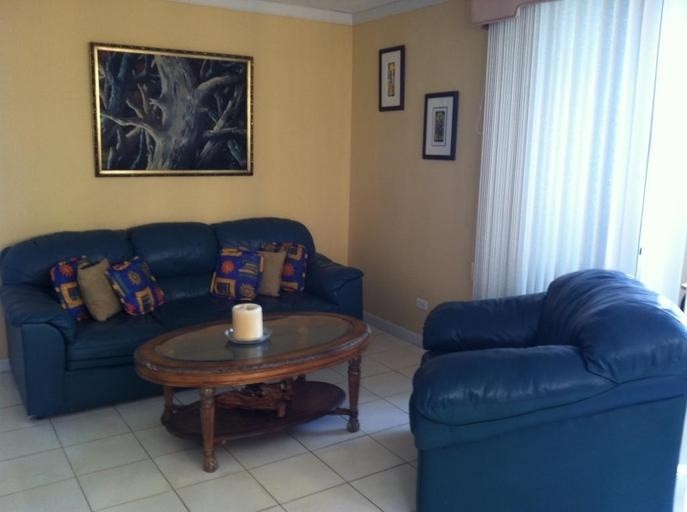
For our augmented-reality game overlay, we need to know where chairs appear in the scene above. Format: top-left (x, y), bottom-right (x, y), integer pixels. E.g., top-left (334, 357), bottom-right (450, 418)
top-left (410, 270), bottom-right (687, 512)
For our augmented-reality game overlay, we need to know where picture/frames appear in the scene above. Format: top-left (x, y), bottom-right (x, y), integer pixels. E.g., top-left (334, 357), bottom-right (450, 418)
top-left (378, 45), bottom-right (405, 112)
top-left (89, 42), bottom-right (254, 177)
top-left (422, 91), bottom-right (459, 160)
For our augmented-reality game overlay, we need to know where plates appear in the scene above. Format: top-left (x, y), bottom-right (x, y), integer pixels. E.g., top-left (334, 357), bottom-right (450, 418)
top-left (224, 327), bottom-right (272, 344)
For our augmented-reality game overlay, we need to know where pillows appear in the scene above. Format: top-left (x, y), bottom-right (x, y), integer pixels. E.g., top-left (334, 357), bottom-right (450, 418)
top-left (50, 254), bottom-right (90, 320)
top-left (253, 251), bottom-right (285, 298)
top-left (104, 255), bottom-right (166, 317)
top-left (77, 259), bottom-right (123, 321)
top-left (260, 242), bottom-right (309, 296)
top-left (209, 246), bottom-right (264, 300)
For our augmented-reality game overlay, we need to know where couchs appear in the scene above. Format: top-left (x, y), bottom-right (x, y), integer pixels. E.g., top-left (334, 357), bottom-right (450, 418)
top-left (0, 217), bottom-right (364, 420)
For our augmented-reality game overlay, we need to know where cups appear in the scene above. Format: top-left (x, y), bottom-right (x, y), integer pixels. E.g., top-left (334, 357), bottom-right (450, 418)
top-left (231, 303), bottom-right (262, 340)
